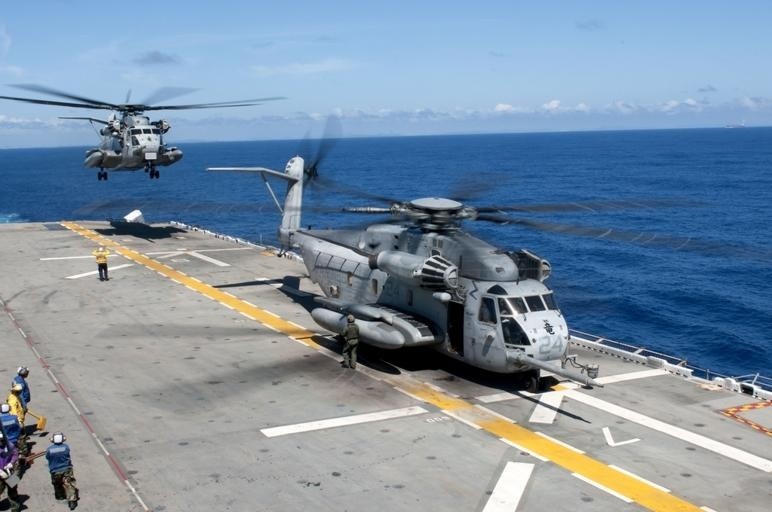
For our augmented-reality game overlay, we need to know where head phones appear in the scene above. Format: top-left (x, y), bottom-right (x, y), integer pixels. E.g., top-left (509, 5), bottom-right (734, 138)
top-left (0, 403), bottom-right (11, 412)
top-left (50, 431), bottom-right (67, 443)
top-left (23, 366), bottom-right (28, 378)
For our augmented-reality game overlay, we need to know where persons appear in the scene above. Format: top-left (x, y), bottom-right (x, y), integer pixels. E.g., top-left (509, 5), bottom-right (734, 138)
top-left (0, 365), bottom-right (80, 512)
top-left (339, 314), bottom-right (360, 369)
top-left (92, 247), bottom-right (111, 281)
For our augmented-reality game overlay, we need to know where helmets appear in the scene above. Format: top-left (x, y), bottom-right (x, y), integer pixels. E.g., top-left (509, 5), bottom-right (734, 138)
top-left (13, 384), bottom-right (23, 391)
top-left (52, 431), bottom-right (64, 444)
top-left (347, 314), bottom-right (355, 323)
top-left (0, 403), bottom-right (11, 414)
top-left (17, 366), bottom-right (29, 375)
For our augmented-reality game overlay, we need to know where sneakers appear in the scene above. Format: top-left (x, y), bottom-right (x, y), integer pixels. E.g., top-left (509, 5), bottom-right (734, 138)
top-left (342, 361), bottom-right (357, 370)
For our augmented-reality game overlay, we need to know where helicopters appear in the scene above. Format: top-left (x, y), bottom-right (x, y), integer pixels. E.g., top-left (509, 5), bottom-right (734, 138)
top-left (1, 80), bottom-right (288, 181)
top-left (206, 114), bottom-right (753, 389)
